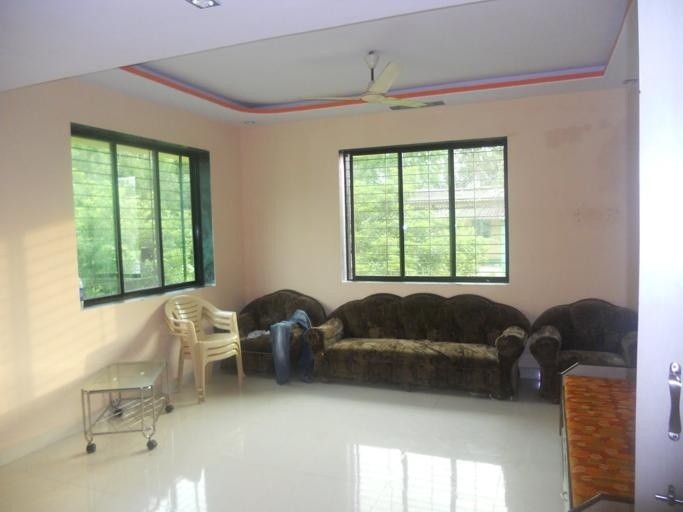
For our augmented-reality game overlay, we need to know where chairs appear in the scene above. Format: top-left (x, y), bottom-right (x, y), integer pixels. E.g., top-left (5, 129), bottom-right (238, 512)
top-left (161, 294), bottom-right (246, 404)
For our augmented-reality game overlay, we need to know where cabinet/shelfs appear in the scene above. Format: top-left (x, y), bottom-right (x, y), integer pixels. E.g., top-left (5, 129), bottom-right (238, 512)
top-left (559, 364), bottom-right (635, 511)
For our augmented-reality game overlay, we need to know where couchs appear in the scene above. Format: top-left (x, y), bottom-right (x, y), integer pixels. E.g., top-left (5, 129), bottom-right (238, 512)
top-left (527, 297), bottom-right (638, 405)
top-left (212, 288), bottom-right (327, 385)
top-left (302, 293), bottom-right (531, 402)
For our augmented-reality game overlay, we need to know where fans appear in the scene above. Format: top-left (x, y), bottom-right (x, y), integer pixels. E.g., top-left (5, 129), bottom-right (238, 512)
top-left (303, 46), bottom-right (430, 110)
